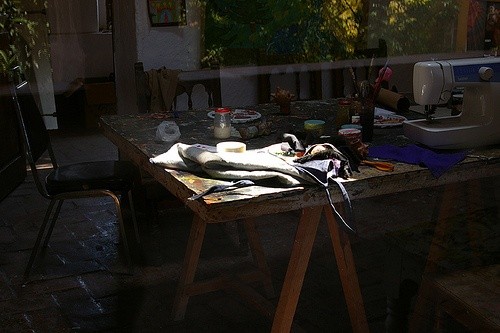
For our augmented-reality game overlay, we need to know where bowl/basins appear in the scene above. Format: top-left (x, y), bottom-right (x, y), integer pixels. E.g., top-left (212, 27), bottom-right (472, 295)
top-left (207, 108), bottom-right (264, 139)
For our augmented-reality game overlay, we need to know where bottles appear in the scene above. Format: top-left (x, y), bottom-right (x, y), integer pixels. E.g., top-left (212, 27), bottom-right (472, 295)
top-left (337, 128), bottom-right (362, 158)
top-left (303, 120), bottom-right (326, 146)
top-left (213, 107), bottom-right (231, 139)
top-left (336, 98), bottom-right (355, 126)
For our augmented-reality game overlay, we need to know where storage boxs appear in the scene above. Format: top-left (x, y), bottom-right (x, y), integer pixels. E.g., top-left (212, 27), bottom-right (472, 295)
top-left (76, 76), bottom-right (115, 128)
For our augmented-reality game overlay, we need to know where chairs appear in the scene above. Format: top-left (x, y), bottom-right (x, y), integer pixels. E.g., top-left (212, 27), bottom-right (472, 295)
top-left (10, 66), bottom-right (147, 287)
top-left (431, 265), bottom-right (500, 333)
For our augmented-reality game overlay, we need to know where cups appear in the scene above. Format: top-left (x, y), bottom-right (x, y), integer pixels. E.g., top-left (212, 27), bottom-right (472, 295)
top-left (216, 141), bottom-right (246, 153)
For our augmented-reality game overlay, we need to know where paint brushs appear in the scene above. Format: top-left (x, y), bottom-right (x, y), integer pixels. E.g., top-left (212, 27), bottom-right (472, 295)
top-left (347, 64), bottom-right (357, 90)
top-left (373, 59), bottom-right (388, 98)
top-left (367, 53), bottom-right (376, 80)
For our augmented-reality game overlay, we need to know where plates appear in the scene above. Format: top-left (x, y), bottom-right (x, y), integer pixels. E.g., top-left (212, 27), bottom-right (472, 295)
top-left (352, 113), bottom-right (408, 127)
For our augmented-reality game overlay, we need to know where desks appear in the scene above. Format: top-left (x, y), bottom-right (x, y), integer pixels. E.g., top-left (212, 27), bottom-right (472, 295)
top-left (97, 92), bottom-right (500, 333)
